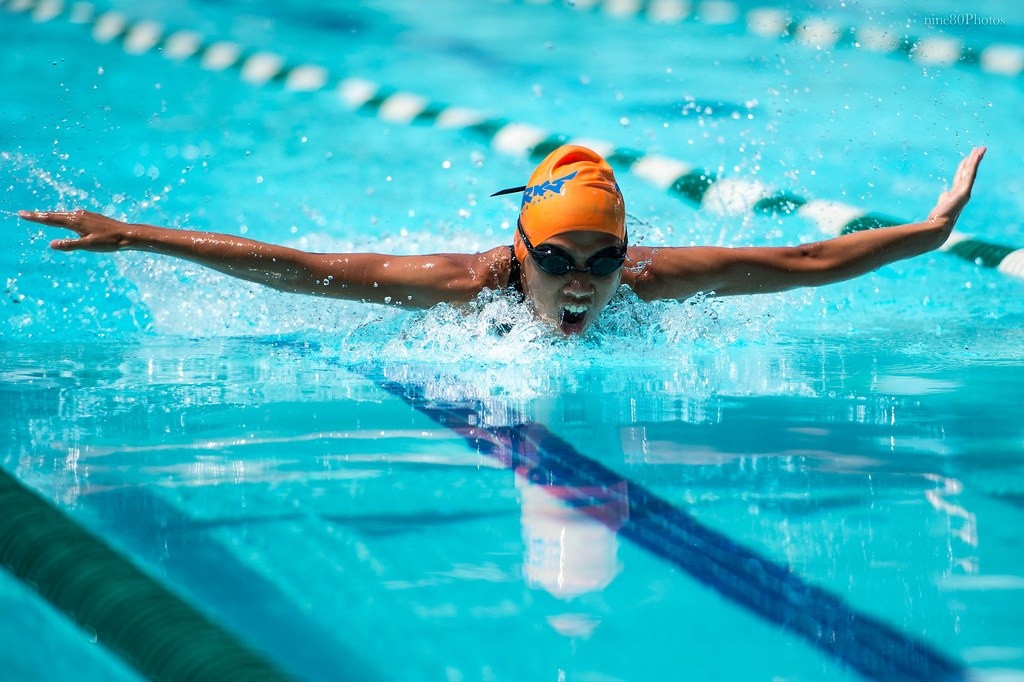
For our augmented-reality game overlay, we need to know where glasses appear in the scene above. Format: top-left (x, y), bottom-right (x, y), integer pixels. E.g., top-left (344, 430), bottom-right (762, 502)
top-left (517, 218), bottom-right (628, 277)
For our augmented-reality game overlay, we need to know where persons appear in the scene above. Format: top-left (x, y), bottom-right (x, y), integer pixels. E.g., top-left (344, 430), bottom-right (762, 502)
top-left (14, 144), bottom-right (987, 340)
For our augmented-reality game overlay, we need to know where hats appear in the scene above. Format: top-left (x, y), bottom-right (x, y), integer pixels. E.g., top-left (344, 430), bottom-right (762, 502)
top-left (514, 143), bottom-right (627, 265)
